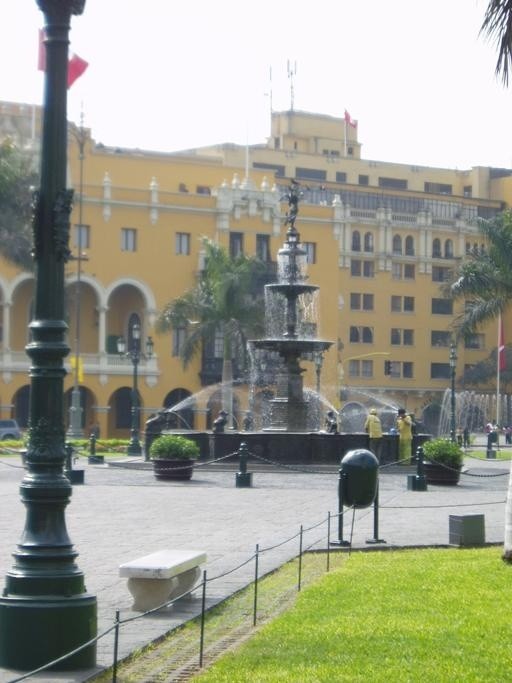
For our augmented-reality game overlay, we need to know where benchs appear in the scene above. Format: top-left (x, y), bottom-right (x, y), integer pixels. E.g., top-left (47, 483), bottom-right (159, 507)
top-left (118, 551), bottom-right (209, 614)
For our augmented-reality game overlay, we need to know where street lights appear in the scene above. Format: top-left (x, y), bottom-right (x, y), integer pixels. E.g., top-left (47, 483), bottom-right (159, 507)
top-left (449, 343), bottom-right (458, 443)
top-left (116, 323), bottom-right (154, 456)
top-left (313, 350), bottom-right (325, 431)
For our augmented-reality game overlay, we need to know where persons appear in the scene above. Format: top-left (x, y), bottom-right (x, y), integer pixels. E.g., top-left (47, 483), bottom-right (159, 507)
top-left (486, 420), bottom-right (512, 445)
top-left (365, 409), bottom-right (383, 463)
top-left (456, 423), bottom-right (470, 448)
top-left (395, 408), bottom-right (413, 466)
top-left (279, 178), bottom-right (303, 230)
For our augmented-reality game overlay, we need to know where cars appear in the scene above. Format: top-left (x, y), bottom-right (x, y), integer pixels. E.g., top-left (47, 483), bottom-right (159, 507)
top-left (0, 419), bottom-right (20, 440)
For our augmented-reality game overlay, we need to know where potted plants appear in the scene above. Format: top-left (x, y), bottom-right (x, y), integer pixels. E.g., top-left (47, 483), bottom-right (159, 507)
top-left (417, 434), bottom-right (465, 485)
top-left (150, 434), bottom-right (202, 480)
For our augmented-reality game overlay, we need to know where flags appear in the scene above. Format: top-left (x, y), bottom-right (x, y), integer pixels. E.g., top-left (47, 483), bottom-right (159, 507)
top-left (499, 313), bottom-right (507, 372)
top-left (37, 29), bottom-right (88, 89)
top-left (345, 110), bottom-right (356, 128)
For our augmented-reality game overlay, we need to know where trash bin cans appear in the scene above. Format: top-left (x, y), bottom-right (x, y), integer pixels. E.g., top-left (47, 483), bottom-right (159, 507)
top-left (416, 433), bottom-right (434, 447)
top-left (487, 432), bottom-right (498, 453)
